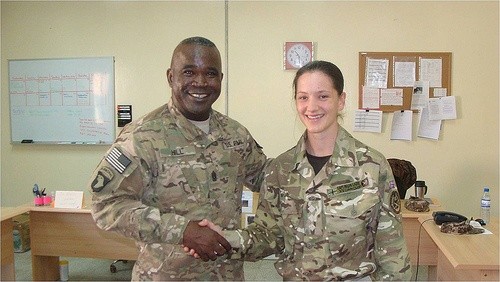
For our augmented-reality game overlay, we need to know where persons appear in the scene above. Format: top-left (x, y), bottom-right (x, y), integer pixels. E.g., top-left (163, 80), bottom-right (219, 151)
top-left (181, 62), bottom-right (412, 282)
top-left (88, 38), bottom-right (274, 282)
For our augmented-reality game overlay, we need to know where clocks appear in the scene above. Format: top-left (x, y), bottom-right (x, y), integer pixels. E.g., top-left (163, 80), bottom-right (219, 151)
top-left (283, 42), bottom-right (312, 70)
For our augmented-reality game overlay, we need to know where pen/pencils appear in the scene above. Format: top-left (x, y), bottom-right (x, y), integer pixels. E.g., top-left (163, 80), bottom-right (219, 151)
top-left (40, 187), bottom-right (47, 195)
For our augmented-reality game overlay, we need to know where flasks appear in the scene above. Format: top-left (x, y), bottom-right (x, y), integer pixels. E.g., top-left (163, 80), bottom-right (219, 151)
top-left (415, 181), bottom-right (427, 199)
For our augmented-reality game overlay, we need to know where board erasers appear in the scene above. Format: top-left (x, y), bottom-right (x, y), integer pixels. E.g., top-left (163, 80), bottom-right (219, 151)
top-left (21, 140), bottom-right (33, 143)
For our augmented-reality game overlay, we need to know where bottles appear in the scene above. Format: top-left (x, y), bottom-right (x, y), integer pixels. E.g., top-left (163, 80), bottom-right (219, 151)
top-left (480, 188), bottom-right (491, 226)
top-left (59, 261), bottom-right (69, 281)
top-left (34, 194), bottom-right (51, 206)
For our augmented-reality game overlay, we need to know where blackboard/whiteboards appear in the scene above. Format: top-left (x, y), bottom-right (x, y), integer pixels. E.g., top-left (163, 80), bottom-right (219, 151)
top-left (8, 56), bottom-right (116, 146)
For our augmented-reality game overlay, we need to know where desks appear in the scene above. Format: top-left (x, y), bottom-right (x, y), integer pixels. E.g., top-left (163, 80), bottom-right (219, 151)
top-left (398, 196), bottom-right (500, 282)
top-left (0, 195), bottom-right (140, 282)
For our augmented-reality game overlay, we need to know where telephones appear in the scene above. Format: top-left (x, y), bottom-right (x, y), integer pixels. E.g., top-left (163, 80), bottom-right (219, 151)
top-left (432, 211), bottom-right (468, 226)
